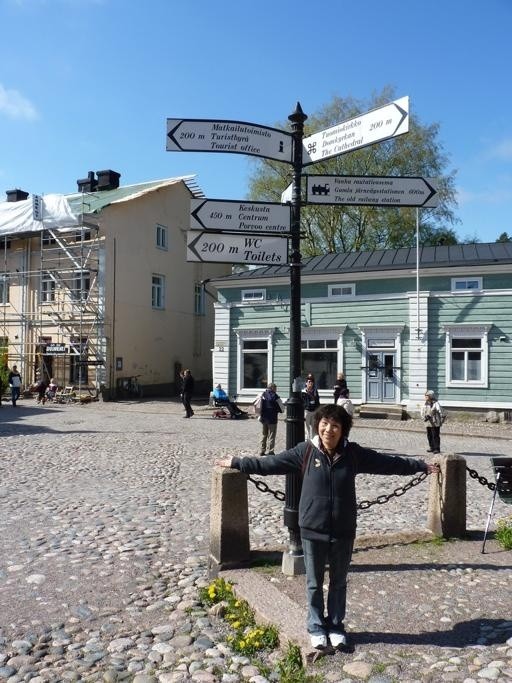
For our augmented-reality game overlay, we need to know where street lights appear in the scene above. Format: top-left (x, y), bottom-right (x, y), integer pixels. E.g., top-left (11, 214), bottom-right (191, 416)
top-left (254, 397), bottom-right (261, 414)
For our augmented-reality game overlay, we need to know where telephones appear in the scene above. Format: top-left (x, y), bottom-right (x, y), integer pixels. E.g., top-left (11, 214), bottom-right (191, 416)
top-left (280, 181), bottom-right (295, 205)
top-left (298, 96), bottom-right (410, 167)
top-left (300, 172), bottom-right (443, 209)
top-left (186, 231), bottom-right (290, 267)
top-left (164, 117), bottom-right (294, 165)
top-left (188, 197), bottom-right (292, 236)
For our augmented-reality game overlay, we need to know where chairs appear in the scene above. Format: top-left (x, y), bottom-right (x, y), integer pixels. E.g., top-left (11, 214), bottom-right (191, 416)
top-left (190, 412), bottom-right (194, 416)
top-left (427, 449), bottom-right (434, 452)
top-left (433, 450), bottom-right (440, 454)
top-left (183, 416), bottom-right (190, 418)
top-left (260, 453), bottom-right (265, 456)
top-left (269, 451), bottom-right (275, 455)
top-left (311, 635), bottom-right (327, 649)
top-left (329, 634), bottom-right (346, 648)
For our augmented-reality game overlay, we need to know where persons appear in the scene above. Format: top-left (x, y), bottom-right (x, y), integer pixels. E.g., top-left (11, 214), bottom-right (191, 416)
top-left (211, 383), bottom-right (248, 417)
top-left (215, 403), bottom-right (443, 650)
top-left (8, 365), bottom-right (22, 407)
top-left (302, 378), bottom-right (320, 441)
top-left (179, 369), bottom-right (195, 419)
top-left (47, 378), bottom-right (58, 401)
top-left (336, 389), bottom-right (354, 418)
top-left (35, 381), bottom-right (46, 405)
top-left (333, 373), bottom-right (347, 404)
top-left (420, 390), bottom-right (442, 453)
top-left (253, 383), bottom-right (285, 456)
